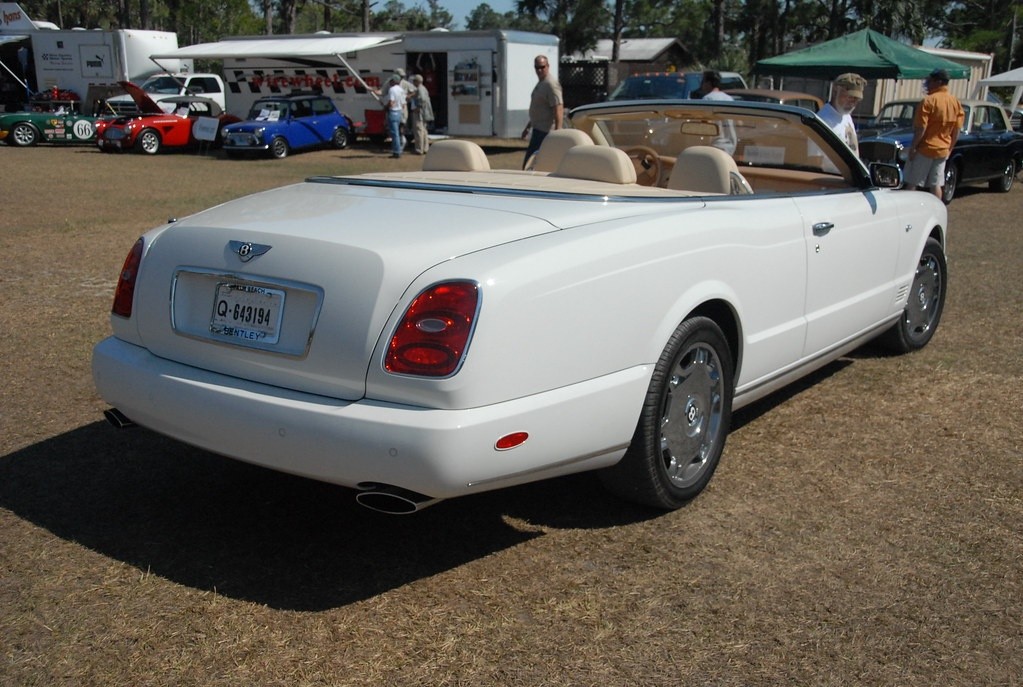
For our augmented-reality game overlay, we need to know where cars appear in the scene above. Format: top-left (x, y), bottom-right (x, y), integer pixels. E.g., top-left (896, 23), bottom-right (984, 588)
top-left (1, 70), bottom-right (352, 159)
top-left (584, 69), bottom-right (1023, 207)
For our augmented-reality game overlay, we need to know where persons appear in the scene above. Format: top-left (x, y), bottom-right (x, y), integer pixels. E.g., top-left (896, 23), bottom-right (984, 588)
top-left (807, 72), bottom-right (867, 174)
top-left (521, 55), bottom-right (564, 170)
top-left (368, 68), bottom-right (435, 158)
top-left (902, 67), bottom-right (965, 199)
top-left (701, 70), bottom-right (745, 156)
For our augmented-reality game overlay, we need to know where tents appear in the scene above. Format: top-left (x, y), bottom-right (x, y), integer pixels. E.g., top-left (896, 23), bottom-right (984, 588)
top-left (756, 25), bottom-right (971, 122)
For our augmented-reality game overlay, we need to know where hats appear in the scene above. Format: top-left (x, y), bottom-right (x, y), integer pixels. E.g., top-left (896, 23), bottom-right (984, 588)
top-left (392, 74), bottom-right (401, 84)
top-left (832, 72), bottom-right (868, 98)
top-left (396, 67), bottom-right (406, 76)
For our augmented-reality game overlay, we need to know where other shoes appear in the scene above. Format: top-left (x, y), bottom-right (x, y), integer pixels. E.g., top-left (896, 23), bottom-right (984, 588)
top-left (409, 150), bottom-right (427, 155)
top-left (387, 152), bottom-right (400, 158)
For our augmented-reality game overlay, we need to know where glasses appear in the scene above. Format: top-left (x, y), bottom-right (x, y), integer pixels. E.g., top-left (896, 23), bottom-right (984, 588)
top-left (534, 65), bottom-right (547, 69)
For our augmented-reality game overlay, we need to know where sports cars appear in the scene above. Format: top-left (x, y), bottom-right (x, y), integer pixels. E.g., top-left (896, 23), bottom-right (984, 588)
top-left (90, 95), bottom-right (953, 515)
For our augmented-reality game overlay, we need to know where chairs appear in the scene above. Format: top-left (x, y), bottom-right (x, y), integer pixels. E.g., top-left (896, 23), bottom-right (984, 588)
top-left (421, 139), bottom-right (490, 170)
top-left (664, 145), bottom-right (751, 196)
top-left (556, 145), bottom-right (636, 184)
top-left (532, 126), bottom-right (595, 172)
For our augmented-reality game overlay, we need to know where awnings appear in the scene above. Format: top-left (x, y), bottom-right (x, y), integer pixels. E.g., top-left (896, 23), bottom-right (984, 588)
top-left (0, 35), bottom-right (35, 95)
top-left (148, 35), bottom-right (403, 114)
